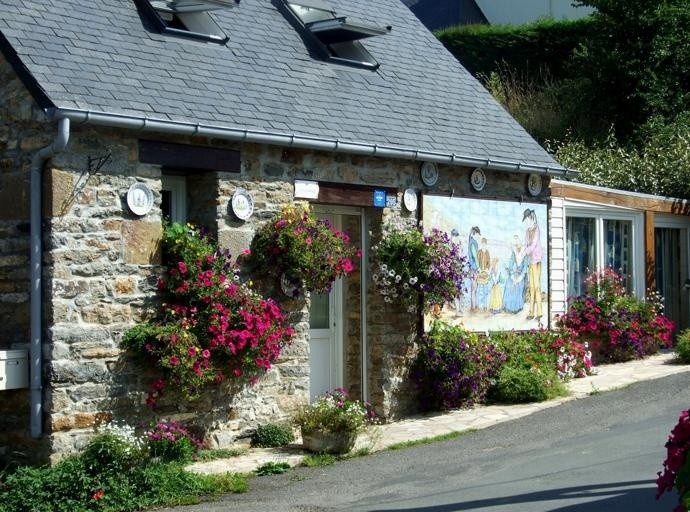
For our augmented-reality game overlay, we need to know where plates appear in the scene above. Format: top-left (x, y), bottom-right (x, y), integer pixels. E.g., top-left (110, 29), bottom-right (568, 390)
top-left (127, 182), bottom-right (154, 216)
top-left (404, 189), bottom-right (418, 213)
top-left (528, 174), bottom-right (543, 197)
top-left (470, 167), bottom-right (487, 192)
top-left (232, 190), bottom-right (255, 221)
top-left (420, 161), bottom-right (439, 187)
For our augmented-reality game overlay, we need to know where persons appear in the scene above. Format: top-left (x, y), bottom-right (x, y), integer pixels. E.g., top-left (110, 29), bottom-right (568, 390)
top-left (475, 237), bottom-right (491, 312)
top-left (488, 256), bottom-right (503, 314)
top-left (521, 208), bottom-right (543, 321)
top-left (468, 226), bottom-right (481, 313)
top-left (502, 233), bottom-right (527, 315)
top-left (446, 229), bottom-right (465, 318)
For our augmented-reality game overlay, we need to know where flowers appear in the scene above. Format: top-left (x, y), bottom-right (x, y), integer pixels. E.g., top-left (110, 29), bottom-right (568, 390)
top-left (369, 201), bottom-right (477, 317)
top-left (288, 388), bottom-right (382, 439)
top-left (411, 320), bottom-right (482, 410)
top-left (120, 202), bottom-right (362, 401)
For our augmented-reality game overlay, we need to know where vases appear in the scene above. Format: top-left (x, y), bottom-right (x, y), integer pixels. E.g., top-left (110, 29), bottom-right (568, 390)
top-left (301, 426), bottom-right (357, 453)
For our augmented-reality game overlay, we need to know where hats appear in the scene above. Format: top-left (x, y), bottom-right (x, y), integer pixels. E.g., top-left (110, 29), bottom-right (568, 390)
top-left (472, 225), bottom-right (482, 236)
top-left (521, 207), bottom-right (535, 222)
